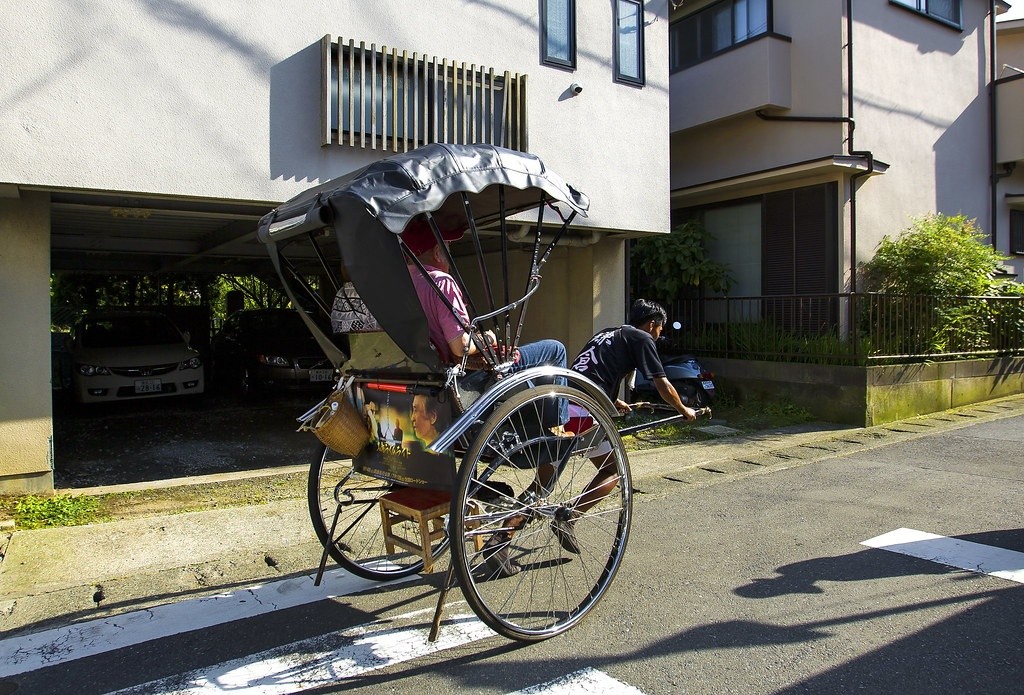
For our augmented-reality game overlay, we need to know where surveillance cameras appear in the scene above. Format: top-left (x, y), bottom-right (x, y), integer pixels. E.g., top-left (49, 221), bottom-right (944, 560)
top-left (571, 83), bottom-right (583, 95)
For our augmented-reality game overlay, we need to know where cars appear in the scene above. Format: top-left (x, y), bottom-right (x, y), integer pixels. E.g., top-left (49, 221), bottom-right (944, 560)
top-left (65, 305), bottom-right (205, 409)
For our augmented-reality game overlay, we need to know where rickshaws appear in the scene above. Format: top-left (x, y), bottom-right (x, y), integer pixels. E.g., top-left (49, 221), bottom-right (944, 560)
top-left (256, 139), bottom-right (714, 648)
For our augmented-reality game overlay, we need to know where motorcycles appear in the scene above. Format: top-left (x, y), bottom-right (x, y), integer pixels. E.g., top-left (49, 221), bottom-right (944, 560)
top-left (629, 317), bottom-right (718, 413)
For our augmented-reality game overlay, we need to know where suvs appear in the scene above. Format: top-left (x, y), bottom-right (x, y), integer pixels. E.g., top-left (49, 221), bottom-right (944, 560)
top-left (208, 307), bottom-right (349, 406)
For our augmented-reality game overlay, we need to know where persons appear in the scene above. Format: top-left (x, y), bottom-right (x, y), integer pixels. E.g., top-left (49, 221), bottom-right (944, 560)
top-left (388, 213), bottom-right (577, 439)
top-left (390, 416), bottom-right (404, 449)
top-left (409, 394), bottom-right (456, 456)
top-left (480, 297), bottom-right (698, 577)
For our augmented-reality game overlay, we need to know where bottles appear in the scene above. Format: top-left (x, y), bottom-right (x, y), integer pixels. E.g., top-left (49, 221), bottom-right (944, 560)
top-left (329, 402), bottom-right (338, 418)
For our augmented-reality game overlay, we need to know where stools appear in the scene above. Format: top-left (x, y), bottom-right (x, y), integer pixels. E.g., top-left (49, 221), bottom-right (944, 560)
top-left (378, 488), bottom-right (484, 573)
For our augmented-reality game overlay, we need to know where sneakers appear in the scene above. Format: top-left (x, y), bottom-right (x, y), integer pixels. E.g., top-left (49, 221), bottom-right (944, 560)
top-left (483, 532), bottom-right (522, 577)
top-left (551, 510), bottom-right (581, 554)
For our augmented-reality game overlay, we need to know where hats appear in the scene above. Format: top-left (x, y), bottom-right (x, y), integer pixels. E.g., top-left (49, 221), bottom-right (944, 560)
top-left (402, 217), bottom-right (465, 259)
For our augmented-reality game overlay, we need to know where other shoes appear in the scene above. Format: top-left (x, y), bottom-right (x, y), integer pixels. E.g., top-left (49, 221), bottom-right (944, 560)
top-left (548, 426), bottom-right (576, 437)
